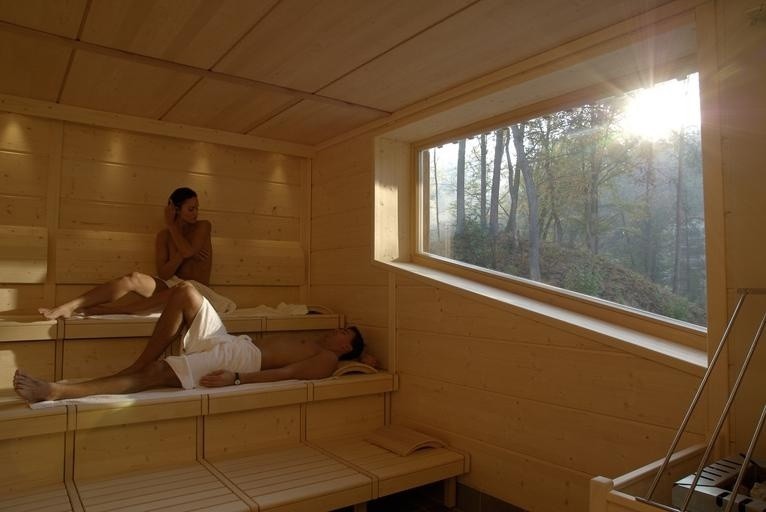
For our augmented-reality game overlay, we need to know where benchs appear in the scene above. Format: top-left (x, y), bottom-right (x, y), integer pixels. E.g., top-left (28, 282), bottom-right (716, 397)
top-left (1, 303), bottom-right (470, 511)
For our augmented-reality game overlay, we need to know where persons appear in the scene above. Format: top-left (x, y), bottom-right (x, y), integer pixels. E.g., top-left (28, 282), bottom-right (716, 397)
top-left (37, 188), bottom-right (214, 320)
top-left (13, 279), bottom-right (378, 403)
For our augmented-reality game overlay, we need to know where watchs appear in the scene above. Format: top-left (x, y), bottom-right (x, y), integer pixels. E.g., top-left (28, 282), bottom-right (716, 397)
top-left (234, 372), bottom-right (241, 385)
